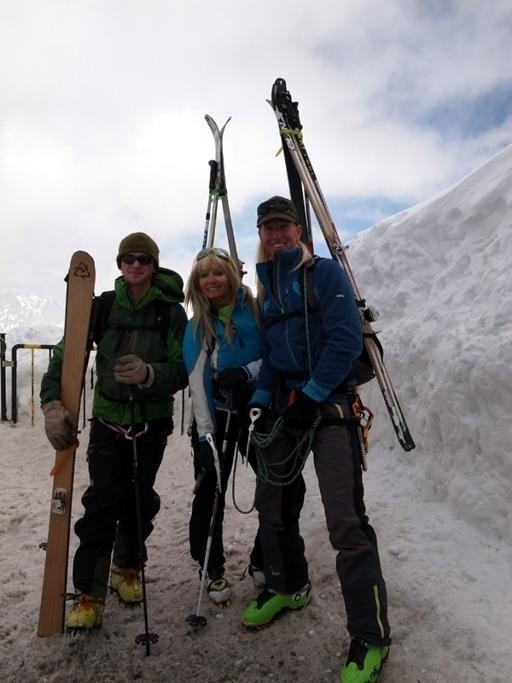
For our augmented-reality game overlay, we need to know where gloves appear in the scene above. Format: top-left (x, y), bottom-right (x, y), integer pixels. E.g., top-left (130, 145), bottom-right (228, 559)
top-left (215, 367), bottom-right (248, 391)
top-left (42, 400), bottom-right (77, 451)
top-left (279, 392), bottom-right (319, 431)
top-left (114, 354), bottom-right (155, 390)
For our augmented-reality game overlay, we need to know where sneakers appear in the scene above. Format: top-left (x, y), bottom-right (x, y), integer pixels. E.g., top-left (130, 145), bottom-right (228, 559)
top-left (198, 568), bottom-right (235, 609)
top-left (339, 636), bottom-right (389, 683)
top-left (240, 581), bottom-right (312, 634)
top-left (109, 561), bottom-right (144, 611)
top-left (59, 592), bottom-right (106, 639)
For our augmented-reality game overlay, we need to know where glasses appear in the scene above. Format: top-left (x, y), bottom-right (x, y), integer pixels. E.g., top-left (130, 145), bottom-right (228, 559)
top-left (258, 201), bottom-right (292, 215)
top-left (119, 255), bottom-right (153, 265)
top-left (197, 248), bottom-right (230, 262)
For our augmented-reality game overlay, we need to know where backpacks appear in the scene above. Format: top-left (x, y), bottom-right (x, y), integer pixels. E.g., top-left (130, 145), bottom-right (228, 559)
top-left (300, 255), bottom-right (383, 387)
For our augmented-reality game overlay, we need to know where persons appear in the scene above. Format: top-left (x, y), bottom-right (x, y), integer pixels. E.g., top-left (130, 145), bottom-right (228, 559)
top-left (241, 194), bottom-right (392, 682)
top-left (180, 245), bottom-right (264, 610)
top-left (40, 230), bottom-right (190, 632)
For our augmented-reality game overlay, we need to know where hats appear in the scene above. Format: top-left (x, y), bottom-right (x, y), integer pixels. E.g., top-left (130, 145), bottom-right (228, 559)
top-left (257, 196), bottom-right (299, 228)
top-left (119, 233), bottom-right (159, 263)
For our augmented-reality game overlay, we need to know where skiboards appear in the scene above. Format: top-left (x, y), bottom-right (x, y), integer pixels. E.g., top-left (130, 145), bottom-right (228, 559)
top-left (38, 250), bottom-right (95, 639)
top-left (266, 79), bottom-right (415, 452)
top-left (203, 114), bottom-right (247, 284)
